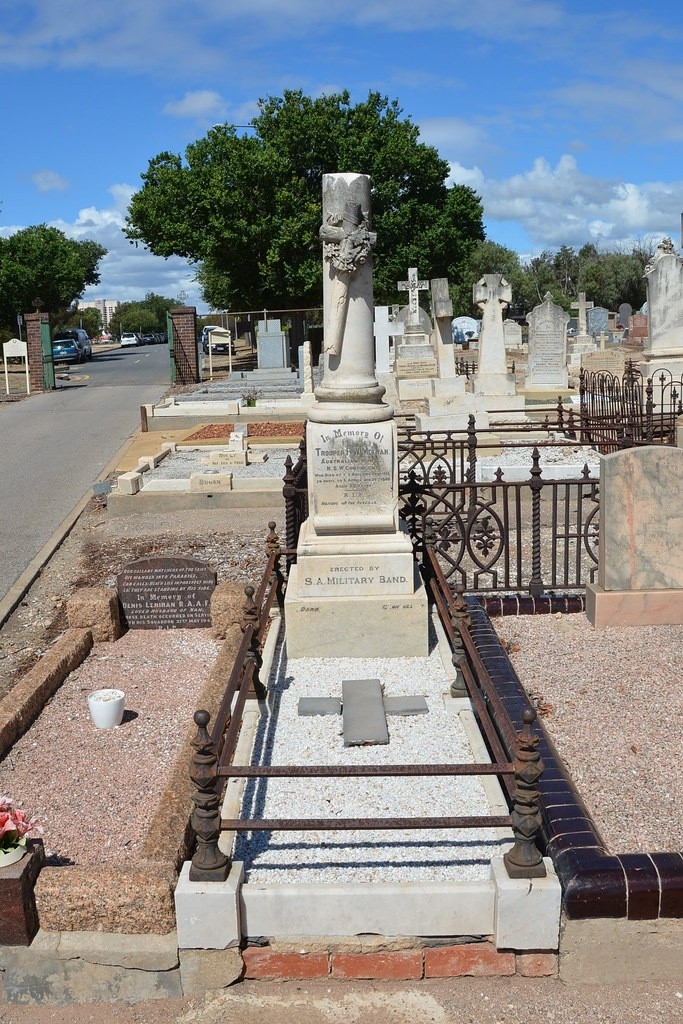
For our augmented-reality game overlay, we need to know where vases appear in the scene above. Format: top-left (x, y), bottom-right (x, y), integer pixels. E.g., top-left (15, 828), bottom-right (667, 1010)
top-left (87, 688), bottom-right (125, 729)
top-left (247, 400), bottom-right (255, 407)
top-left (0, 844), bottom-right (27, 868)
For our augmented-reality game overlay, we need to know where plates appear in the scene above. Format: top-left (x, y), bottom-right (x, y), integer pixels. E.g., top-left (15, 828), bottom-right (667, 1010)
top-left (204, 469), bottom-right (219, 473)
top-left (222, 449), bottom-right (235, 452)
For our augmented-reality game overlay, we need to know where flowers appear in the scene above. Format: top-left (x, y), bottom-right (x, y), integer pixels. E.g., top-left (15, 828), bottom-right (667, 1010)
top-left (616, 323), bottom-right (625, 329)
top-left (241, 390), bottom-right (258, 401)
top-left (0, 796), bottom-right (44, 855)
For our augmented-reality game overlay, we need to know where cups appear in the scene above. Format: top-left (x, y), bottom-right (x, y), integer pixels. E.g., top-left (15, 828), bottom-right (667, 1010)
top-left (88, 689), bottom-right (125, 728)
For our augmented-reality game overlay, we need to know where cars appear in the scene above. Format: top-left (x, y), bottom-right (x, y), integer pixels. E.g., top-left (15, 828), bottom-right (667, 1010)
top-left (55, 330), bottom-right (94, 363)
top-left (202, 325), bottom-right (236, 355)
top-left (52, 339), bottom-right (83, 364)
top-left (121, 332), bottom-right (166, 347)
